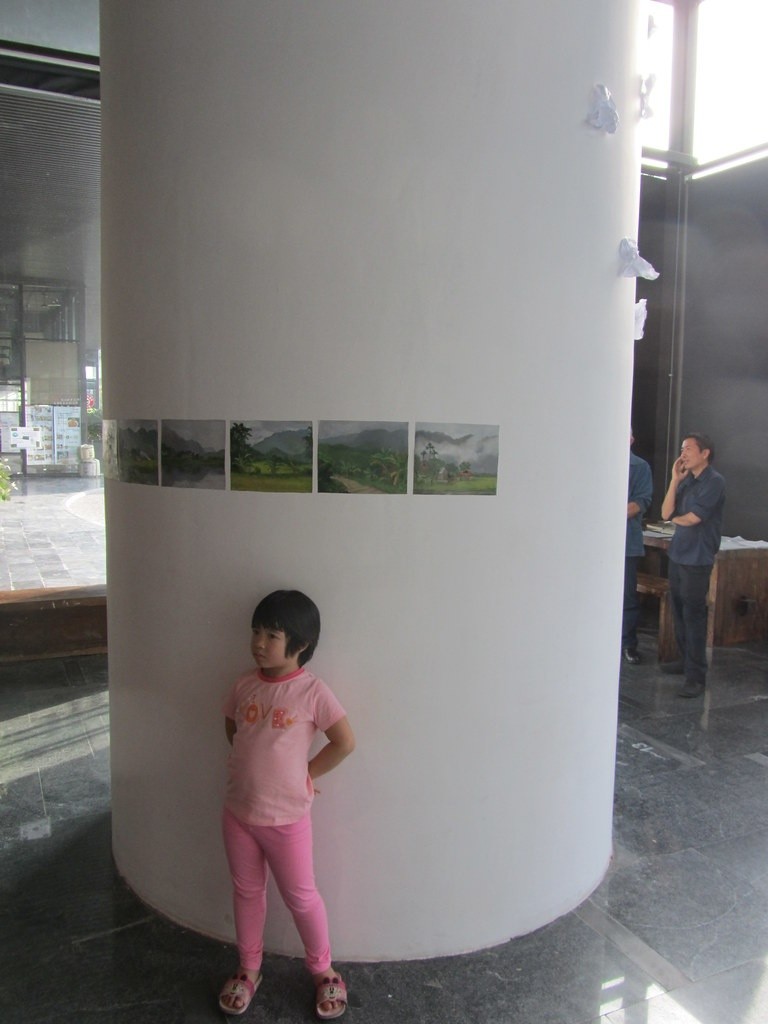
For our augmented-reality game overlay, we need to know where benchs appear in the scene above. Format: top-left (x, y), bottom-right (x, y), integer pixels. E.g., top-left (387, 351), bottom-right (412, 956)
top-left (633, 572), bottom-right (680, 663)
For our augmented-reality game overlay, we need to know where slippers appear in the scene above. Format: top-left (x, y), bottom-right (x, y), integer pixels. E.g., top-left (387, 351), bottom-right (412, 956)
top-left (315, 972), bottom-right (348, 1019)
top-left (219, 969), bottom-right (263, 1014)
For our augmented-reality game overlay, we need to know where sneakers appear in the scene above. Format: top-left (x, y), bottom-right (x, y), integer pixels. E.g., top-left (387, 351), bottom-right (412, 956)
top-left (660, 662), bottom-right (684, 673)
top-left (678, 672), bottom-right (707, 698)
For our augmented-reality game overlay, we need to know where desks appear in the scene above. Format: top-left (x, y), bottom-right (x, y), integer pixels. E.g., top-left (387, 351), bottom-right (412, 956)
top-left (640, 525), bottom-right (768, 643)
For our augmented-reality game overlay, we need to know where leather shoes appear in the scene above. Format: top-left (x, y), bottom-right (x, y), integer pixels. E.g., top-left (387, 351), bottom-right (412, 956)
top-left (623, 647), bottom-right (642, 663)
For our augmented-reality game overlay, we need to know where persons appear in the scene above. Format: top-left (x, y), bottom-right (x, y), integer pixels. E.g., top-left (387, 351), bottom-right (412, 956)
top-left (219, 590), bottom-right (354, 1020)
top-left (619, 427), bottom-right (653, 664)
top-left (660, 431), bottom-right (726, 698)
